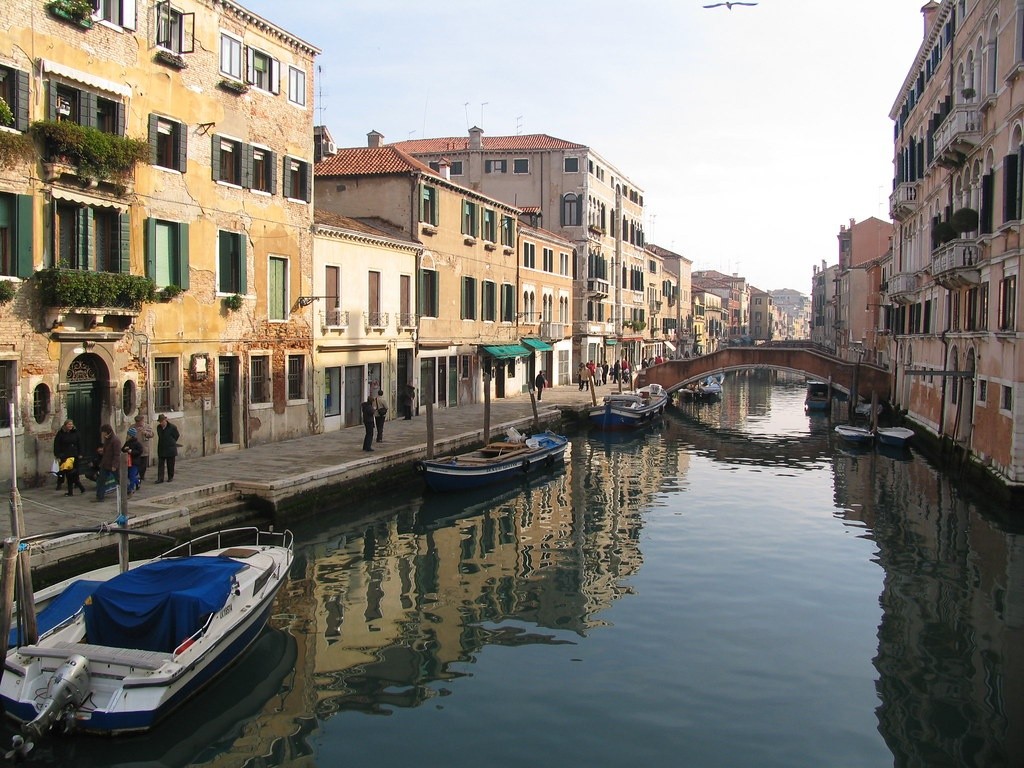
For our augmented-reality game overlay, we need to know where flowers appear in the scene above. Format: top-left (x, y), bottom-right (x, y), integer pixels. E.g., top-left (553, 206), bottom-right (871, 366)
top-left (47, 0), bottom-right (95, 19)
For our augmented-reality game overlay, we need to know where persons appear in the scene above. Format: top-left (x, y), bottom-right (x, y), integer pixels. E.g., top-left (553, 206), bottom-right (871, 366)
top-left (601, 361), bottom-right (608, 385)
top-left (596, 363), bottom-right (603, 386)
top-left (121, 428), bottom-right (142, 498)
top-left (373, 390), bottom-right (388, 443)
top-left (622, 360), bottom-right (630, 383)
top-left (536, 371), bottom-right (545, 400)
top-left (685, 351), bottom-right (689, 358)
top-left (155, 414), bottom-right (180, 483)
top-left (54, 419), bottom-right (85, 496)
top-left (90, 424), bottom-right (133, 502)
top-left (360, 396), bottom-right (375, 452)
top-left (403, 383), bottom-right (415, 420)
top-left (89, 442), bottom-right (103, 479)
top-left (129, 415), bottom-right (154, 480)
top-left (609, 360), bottom-right (620, 384)
top-left (576, 360), bottom-right (596, 391)
top-left (642, 356), bottom-right (662, 369)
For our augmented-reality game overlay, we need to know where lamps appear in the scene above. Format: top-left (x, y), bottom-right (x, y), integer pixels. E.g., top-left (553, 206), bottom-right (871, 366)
top-left (517, 311), bottom-right (542, 319)
top-left (865, 304), bottom-right (891, 313)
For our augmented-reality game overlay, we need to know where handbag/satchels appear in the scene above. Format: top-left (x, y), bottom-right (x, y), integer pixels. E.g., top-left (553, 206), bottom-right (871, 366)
top-left (378, 408), bottom-right (387, 416)
top-left (51, 459), bottom-right (59, 476)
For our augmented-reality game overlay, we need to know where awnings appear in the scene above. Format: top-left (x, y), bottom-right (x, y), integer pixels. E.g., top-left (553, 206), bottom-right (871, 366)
top-left (482, 345), bottom-right (530, 361)
top-left (664, 341), bottom-right (676, 351)
top-left (522, 338), bottom-right (553, 351)
top-left (731, 337), bottom-right (752, 343)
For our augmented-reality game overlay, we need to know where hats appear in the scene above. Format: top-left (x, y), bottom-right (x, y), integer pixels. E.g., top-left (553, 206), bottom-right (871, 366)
top-left (128, 428), bottom-right (137, 437)
top-left (157, 414), bottom-right (167, 421)
top-left (134, 415), bottom-right (144, 421)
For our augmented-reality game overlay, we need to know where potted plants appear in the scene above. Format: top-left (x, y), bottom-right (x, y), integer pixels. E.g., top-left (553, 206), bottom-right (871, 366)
top-left (226, 294), bottom-right (244, 313)
top-left (32, 119), bottom-right (157, 198)
top-left (589, 224), bottom-right (607, 238)
top-left (961, 89), bottom-right (975, 100)
top-left (160, 285), bottom-right (181, 298)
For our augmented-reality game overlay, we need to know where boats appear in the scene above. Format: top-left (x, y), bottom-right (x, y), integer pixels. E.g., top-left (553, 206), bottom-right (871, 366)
top-left (876, 425), bottom-right (915, 448)
top-left (0, 523), bottom-right (297, 762)
top-left (855, 403), bottom-right (883, 419)
top-left (834, 424), bottom-right (875, 442)
top-left (414, 427), bottom-right (568, 492)
top-left (677, 374), bottom-right (725, 399)
top-left (588, 383), bottom-right (668, 429)
top-left (804, 379), bottom-right (832, 412)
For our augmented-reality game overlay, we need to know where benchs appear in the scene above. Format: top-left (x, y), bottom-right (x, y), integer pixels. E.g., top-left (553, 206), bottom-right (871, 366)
top-left (486, 446), bottom-right (529, 461)
top-left (455, 456), bottom-right (487, 462)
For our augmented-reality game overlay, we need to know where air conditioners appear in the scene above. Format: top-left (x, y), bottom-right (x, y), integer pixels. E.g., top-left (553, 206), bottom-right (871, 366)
top-left (323, 141), bottom-right (337, 156)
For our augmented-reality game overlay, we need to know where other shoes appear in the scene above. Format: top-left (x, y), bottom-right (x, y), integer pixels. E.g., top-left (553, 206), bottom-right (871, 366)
top-left (56, 486), bottom-right (62, 490)
top-left (137, 479), bottom-right (141, 490)
top-left (65, 493), bottom-right (73, 496)
top-left (90, 498), bottom-right (104, 502)
top-left (155, 479), bottom-right (163, 483)
top-left (127, 490), bottom-right (134, 499)
top-left (168, 478), bottom-right (173, 482)
top-left (81, 489), bottom-right (85, 493)
